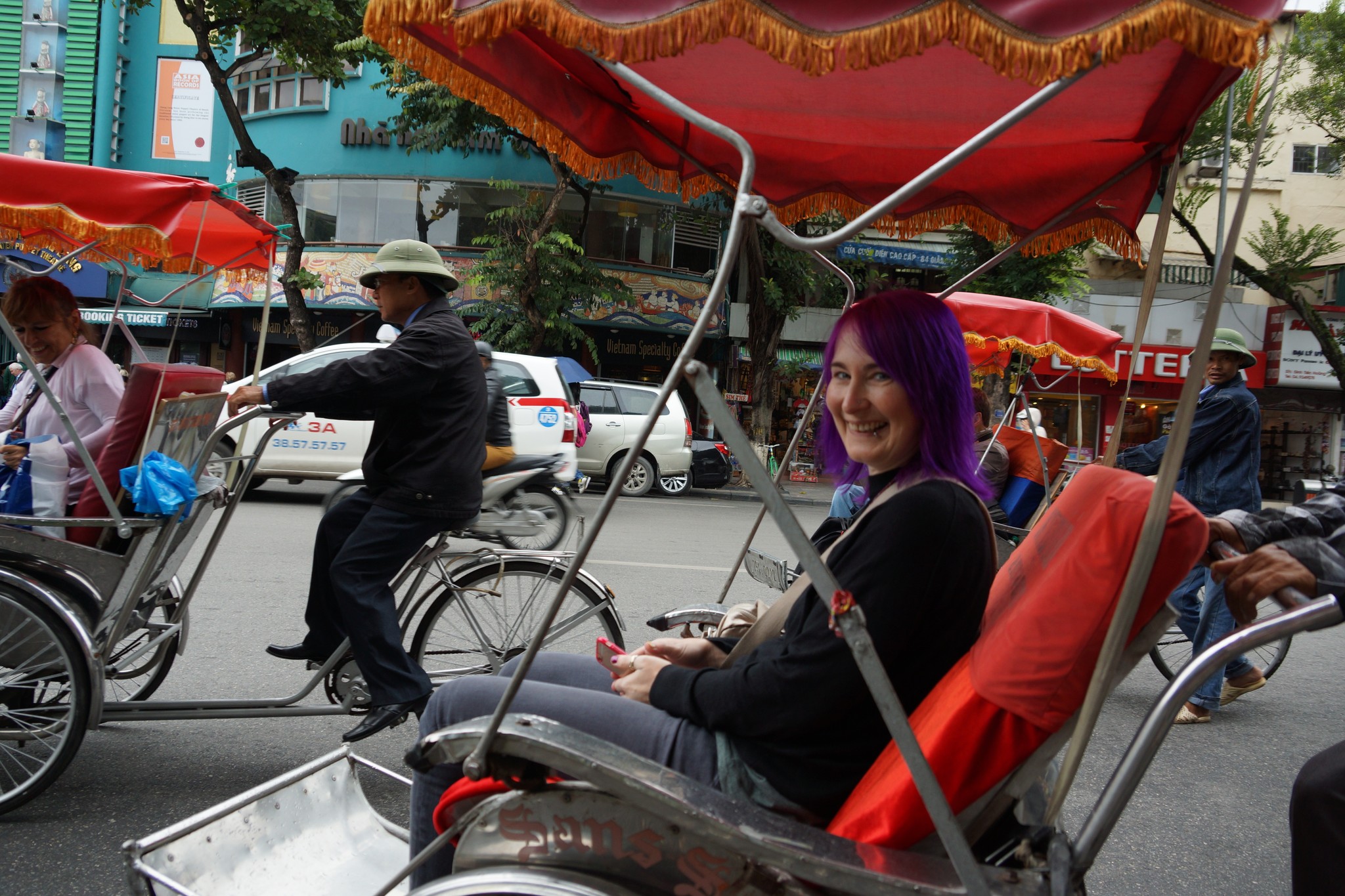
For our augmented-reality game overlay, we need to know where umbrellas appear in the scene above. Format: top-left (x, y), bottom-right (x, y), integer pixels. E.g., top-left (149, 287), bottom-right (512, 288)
top-left (547, 356), bottom-right (596, 386)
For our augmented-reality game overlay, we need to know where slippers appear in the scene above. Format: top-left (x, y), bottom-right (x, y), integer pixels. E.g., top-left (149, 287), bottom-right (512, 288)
top-left (1218, 677), bottom-right (1266, 707)
top-left (1176, 704), bottom-right (1212, 722)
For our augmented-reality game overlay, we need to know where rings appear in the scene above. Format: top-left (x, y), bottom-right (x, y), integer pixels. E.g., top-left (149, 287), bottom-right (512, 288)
top-left (629, 654), bottom-right (639, 671)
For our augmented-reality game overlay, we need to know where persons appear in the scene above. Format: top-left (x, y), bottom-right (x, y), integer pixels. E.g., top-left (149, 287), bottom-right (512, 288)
top-left (226, 237), bottom-right (492, 742)
top-left (0, 275), bottom-right (129, 521)
top-left (9, 362), bottom-right (26, 396)
top-left (1088, 326), bottom-right (1268, 729)
top-left (3, 352), bottom-right (27, 401)
top-left (828, 384), bottom-right (1012, 518)
top-left (550, 383), bottom-right (591, 495)
top-left (471, 338), bottom-right (515, 470)
top-left (1194, 478), bottom-right (1345, 896)
top-left (1016, 406), bottom-right (1049, 439)
top-left (404, 287), bottom-right (1003, 896)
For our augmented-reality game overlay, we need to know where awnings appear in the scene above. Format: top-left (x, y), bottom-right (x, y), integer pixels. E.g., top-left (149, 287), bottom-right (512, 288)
top-left (75, 303), bottom-right (215, 333)
top-left (737, 346), bottom-right (826, 372)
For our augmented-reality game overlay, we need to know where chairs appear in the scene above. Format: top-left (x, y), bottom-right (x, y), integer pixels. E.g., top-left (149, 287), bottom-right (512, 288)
top-left (79, 362), bottom-right (233, 556)
top-left (432, 453), bottom-right (1207, 882)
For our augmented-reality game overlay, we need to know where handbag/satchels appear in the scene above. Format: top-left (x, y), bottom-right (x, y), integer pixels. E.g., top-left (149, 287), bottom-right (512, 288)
top-left (1, 433), bottom-right (68, 541)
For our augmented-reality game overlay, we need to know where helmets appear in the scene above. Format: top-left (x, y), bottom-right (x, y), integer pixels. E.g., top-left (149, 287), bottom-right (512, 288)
top-left (474, 341), bottom-right (492, 359)
top-left (1018, 407), bottom-right (1041, 427)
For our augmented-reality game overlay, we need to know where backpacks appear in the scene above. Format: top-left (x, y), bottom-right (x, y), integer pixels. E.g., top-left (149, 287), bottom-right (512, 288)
top-left (579, 401), bottom-right (592, 435)
top-left (575, 412), bottom-right (586, 448)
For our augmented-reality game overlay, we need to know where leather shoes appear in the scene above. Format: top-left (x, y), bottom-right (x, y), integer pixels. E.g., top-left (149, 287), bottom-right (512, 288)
top-left (265, 643), bottom-right (327, 659)
top-left (344, 689), bottom-right (432, 742)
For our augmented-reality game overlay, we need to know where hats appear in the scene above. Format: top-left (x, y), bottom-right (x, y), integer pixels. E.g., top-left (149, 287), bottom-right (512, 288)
top-left (360, 240), bottom-right (458, 292)
top-left (1190, 328), bottom-right (1256, 369)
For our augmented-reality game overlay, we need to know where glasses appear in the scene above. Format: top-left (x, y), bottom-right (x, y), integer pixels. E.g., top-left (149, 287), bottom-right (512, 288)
top-left (373, 278), bottom-right (382, 288)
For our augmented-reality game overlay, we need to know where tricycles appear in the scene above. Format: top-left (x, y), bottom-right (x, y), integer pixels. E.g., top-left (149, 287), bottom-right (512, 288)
top-left (0, 3), bottom-right (1345, 896)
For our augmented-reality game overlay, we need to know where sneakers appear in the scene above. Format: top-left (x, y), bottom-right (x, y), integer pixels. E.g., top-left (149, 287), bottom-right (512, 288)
top-left (577, 476), bottom-right (591, 494)
top-left (551, 487), bottom-right (570, 495)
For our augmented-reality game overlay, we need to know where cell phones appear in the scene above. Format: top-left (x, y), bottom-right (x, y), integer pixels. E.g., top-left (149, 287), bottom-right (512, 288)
top-left (595, 636), bottom-right (628, 678)
